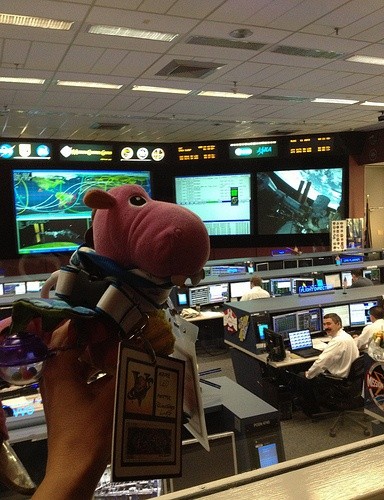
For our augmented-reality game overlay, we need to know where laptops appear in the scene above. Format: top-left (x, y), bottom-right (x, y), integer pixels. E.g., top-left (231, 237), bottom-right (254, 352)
top-left (288, 329), bottom-right (324, 358)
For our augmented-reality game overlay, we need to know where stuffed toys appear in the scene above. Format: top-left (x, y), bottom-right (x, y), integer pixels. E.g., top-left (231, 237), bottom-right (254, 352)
top-left (25, 184), bottom-right (210, 363)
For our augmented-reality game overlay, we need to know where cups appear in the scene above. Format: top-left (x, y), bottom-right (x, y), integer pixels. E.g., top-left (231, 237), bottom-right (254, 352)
top-left (195, 304), bottom-right (201, 313)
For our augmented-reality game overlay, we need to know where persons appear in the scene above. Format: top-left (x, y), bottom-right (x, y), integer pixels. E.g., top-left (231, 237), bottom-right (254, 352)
top-left (354, 306), bottom-right (384, 362)
top-left (29, 320), bottom-right (116, 500)
top-left (346, 269), bottom-right (373, 289)
top-left (240, 276), bottom-right (270, 301)
top-left (296, 313), bottom-right (360, 418)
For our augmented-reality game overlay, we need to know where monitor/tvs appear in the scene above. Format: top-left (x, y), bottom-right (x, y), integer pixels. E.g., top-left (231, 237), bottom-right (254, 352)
top-left (271, 300), bottom-right (379, 345)
top-left (263, 327), bottom-right (286, 362)
top-left (177, 253), bottom-right (374, 309)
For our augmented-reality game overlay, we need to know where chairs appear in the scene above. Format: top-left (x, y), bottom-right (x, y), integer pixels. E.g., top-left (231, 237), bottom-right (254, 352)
top-left (308, 352), bottom-right (373, 438)
